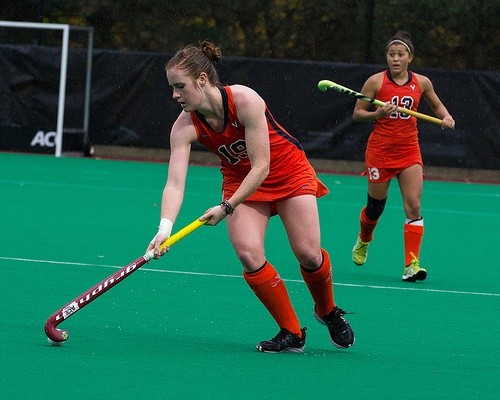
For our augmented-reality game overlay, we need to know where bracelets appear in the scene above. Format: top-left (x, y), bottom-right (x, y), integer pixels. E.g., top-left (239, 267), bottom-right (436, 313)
top-left (220, 201), bottom-right (235, 216)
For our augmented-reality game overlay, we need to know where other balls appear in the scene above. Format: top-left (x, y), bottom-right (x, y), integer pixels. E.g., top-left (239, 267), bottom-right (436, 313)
top-left (47, 337), bottom-right (61, 346)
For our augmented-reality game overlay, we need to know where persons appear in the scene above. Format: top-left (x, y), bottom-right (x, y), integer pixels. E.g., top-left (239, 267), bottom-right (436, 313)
top-left (353, 35), bottom-right (455, 282)
top-left (145, 42), bottom-right (355, 354)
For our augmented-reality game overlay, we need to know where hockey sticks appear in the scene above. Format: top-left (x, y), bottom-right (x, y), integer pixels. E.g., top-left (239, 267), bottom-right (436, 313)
top-left (44, 213), bottom-right (211, 343)
top-left (318, 79), bottom-right (455, 130)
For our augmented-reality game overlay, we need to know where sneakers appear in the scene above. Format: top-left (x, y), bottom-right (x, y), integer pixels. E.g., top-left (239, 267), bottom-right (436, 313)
top-left (256, 327), bottom-right (308, 354)
top-left (352, 235), bottom-right (369, 265)
top-left (402, 252), bottom-right (427, 282)
top-left (312, 304), bottom-right (355, 349)
